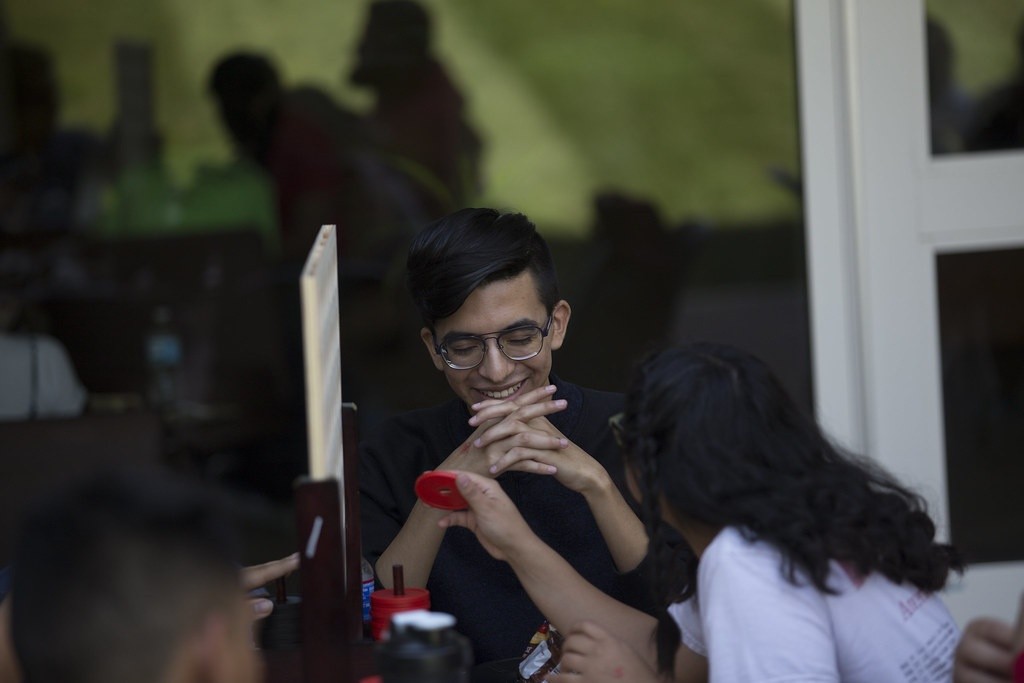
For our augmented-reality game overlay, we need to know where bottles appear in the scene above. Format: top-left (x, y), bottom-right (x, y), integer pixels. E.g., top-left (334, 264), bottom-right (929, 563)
top-left (376, 611), bottom-right (470, 683)
top-left (371, 564), bottom-right (430, 642)
top-left (361, 555), bottom-right (374, 636)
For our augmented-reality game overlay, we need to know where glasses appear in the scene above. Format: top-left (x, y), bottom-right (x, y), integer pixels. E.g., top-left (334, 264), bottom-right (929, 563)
top-left (431, 317), bottom-right (553, 370)
top-left (608, 412), bottom-right (627, 446)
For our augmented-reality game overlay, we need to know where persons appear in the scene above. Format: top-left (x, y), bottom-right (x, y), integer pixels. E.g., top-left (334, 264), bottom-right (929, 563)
top-left (205, 0), bottom-right (481, 260)
top-left (357, 208), bottom-right (695, 683)
top-left (1, 39), bottom-right (157, 229)
top-left (0, 281), bottom-right (90, 420)
top-left (0, 498), bottom-right (305, 683)
top-left (425, 340), bottom-right (1024, 682)
